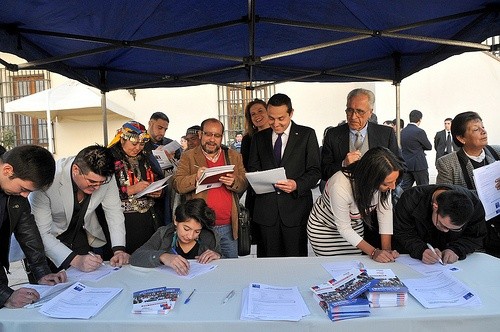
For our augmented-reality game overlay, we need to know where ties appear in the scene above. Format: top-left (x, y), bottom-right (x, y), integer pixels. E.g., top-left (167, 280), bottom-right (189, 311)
top-left (354, 132), bottom-right (364, 150)
top-left (447, 132), bottom-right (452, 154)
top-left (273, 133), bottom-right (284, 162)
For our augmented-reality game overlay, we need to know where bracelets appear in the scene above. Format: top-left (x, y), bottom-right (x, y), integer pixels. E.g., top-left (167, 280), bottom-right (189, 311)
top-left (370, 248), bottom-right (379, 259)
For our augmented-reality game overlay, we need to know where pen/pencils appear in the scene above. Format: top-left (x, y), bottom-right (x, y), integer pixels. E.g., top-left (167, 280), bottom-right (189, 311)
top-left (194, 164), bottom-right (199, 168)
top-left (427, 243), bottom-right (444, 265)
top-left (184, 289), bottom-right (196, 304)
top-left (172, 248), bottom-right (178, 255)
top-left (224, 290), bottom-right (235, 303)
top-left (88, 251), bottom-right (106, 266)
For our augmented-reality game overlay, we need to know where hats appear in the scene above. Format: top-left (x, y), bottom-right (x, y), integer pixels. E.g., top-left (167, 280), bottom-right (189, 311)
top-left (186, 125), bottom-right (201, 136)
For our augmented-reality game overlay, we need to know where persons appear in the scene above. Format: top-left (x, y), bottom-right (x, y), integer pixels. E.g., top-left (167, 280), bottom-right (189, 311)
top-left (239, 93), bottom-right (321, 258)
top-left (392, 119), bottom-right (404, 134)
top-left (232, 133), bottom-right (243, 150)
top-left (0, 145), bottom-right (67, 308)
top-left (27, 143), bottom-right (130, 274)
top-left (434, 112), bottom-right (500, 258)
top-left (145, 112), bottom-right (182, 227)
top-left (306, 146), bottom-right (406, 262)
top-left (400, 111), bottom-right (432, 188)
top-left (319, 88), bottom-right (406, 254)
top-left (173, 118), bottom-right (246, 256)
top-left (391, 183), bottom-right (486, 265)
top-left (383, 120), bottom-right (392, 125)
top-left (129, 198), bottom-right (226, 276)
top-left (107, 120), bottom-right (168, 255)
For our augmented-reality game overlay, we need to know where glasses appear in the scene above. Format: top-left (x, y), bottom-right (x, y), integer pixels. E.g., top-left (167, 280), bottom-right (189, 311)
top-left (345, 108), bottom-right (371, 116)
top-left (436, 214), bottom-right (462, 232)
top-left (76, 163), bottom-right (113, 188)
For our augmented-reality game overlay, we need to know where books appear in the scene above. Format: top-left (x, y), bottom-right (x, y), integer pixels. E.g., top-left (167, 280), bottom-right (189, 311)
top-left (131, 286), bottom-right (182, 313)
top-left (136, 184), bottom-right (169, 201)
top-left (311, 269), bottom-right (409, 321)
top-left (199, 170), bottom-right (234, 185)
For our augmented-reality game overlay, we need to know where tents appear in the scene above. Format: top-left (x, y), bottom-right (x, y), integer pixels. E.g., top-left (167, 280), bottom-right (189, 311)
top-left (0, 0), bottom-right (500, 149)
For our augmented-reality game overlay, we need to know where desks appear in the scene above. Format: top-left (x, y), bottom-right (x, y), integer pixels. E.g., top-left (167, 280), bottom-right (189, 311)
top-left (0, 254), bottom-right (500, 332)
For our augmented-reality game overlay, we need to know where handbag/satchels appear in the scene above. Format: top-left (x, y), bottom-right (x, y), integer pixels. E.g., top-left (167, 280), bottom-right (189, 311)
top-left (237, 203), bottom-right (251, 257)
